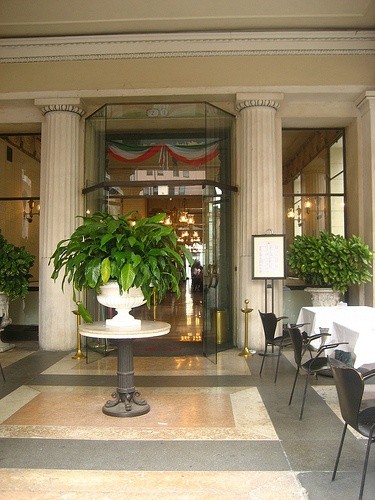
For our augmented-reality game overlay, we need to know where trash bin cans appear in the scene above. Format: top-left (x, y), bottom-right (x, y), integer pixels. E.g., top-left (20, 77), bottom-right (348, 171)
top-left (210, 307), bottom-right (229, 345)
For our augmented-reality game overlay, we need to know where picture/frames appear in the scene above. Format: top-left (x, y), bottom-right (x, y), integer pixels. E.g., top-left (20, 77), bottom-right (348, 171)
top-left (251, 234), bottom-right (285, 280)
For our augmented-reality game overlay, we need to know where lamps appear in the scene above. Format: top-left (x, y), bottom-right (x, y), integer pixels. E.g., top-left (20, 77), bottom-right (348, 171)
top-left (24, 200), bottom-right (40, 223)
top-left (287, 205), bottom-right (303, 227)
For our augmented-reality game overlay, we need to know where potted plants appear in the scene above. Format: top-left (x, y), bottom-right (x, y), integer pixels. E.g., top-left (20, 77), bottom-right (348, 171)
top-left (49, 210), bottom-right (193, 328)
top-left (286, 229), bottom-right (373, 306)
top-left (0, 228), bottom-right (36, 352)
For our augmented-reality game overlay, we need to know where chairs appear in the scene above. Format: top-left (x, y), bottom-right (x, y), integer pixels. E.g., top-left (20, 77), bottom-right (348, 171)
top-left (258, 309), bottom-right (292, 384)
top-left (289, 323), bottom-right (349, 420)
top-left (328, 356), bottom-right (375, 500)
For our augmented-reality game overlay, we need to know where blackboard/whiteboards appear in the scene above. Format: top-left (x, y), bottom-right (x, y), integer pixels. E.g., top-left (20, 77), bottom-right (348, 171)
top-left (251, 234), bottom-right (287, 280)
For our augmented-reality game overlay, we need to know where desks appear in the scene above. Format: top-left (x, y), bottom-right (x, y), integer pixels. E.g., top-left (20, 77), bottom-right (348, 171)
top-left (333, 321), bottom-right (375, 367)
top-left (79, 321), bottom-right (171, 417)
top-left (295, 307), bottom-right (375, 349)
